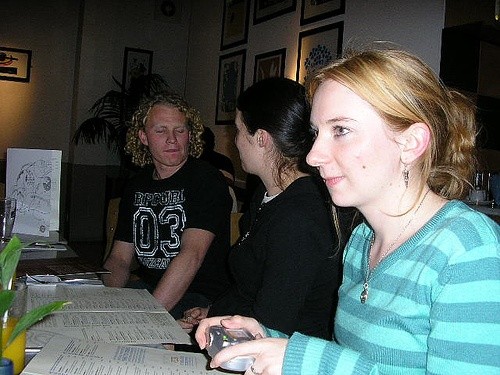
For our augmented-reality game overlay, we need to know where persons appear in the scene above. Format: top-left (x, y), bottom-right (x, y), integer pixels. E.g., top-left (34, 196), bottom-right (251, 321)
top-left (191, 38), bottom-right (500, 375)
top-left (106, 91), bottom-right (237, 317)
top-left (168, 76), bottom-right (341, 353)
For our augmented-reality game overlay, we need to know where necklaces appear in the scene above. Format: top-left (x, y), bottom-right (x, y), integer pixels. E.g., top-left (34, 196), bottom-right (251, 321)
top-left (360, 188), bottom-right (429, 302)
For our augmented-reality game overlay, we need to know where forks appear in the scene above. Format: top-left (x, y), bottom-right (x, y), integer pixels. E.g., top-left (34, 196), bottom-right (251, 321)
top-left (25, 272), bottom-right (103, 286)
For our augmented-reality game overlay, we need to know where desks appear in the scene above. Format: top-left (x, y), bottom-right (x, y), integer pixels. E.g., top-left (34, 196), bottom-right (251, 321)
top-left (0, 232), bottom-right (198, 374)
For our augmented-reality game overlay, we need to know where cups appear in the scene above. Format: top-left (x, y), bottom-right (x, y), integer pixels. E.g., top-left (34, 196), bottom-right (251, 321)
top-left (0, 198), bottom-right (16, 243)
top-left (0, 280), bottom-right (28, 375)
top-left (469, 170), bottom-right (496, 202)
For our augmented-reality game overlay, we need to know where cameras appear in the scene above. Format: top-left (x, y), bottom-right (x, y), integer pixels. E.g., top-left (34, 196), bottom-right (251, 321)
top-left (205, 326), bottom-right (256, 371)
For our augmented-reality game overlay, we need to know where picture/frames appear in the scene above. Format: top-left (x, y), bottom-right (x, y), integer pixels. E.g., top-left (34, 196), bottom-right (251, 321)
top-left (300, 0), bottom-right (346, 26)
top-left (297, 20), bottom-right (345, 86)
top-left (252, 47), bottom-right (287, 84)
top-left (0, 46), bottom-right (33, 81)
top-left (215, 49), bottom-right (247, 125)
top-left (220, 0), bottom-right (251, 51)
top-left (253, 0), bottom-right (298, 25)
top-left (122, 47), bottom-right (154, 99)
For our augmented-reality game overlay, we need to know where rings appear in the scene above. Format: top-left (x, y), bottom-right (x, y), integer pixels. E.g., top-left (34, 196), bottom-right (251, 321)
top-left (250, 366), bottom-right (262, 375)
